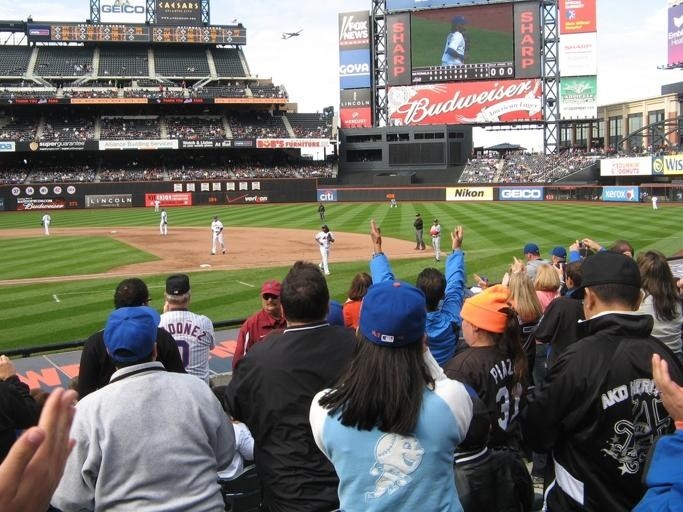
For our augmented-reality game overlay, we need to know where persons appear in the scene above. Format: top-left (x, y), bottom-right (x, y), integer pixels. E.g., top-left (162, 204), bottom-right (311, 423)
top-left (150, 199), bottom-right (162, 213)
top-left (157, 208), bottom-right (170, 237)
top-left (649, 192), bottom-right (661, 210)
top-left (316, 200), bottom-right (329, 221)
top-left (388, 195), bottom-right (399, 207)
top-left (441, 16), bottom-right (474, 65)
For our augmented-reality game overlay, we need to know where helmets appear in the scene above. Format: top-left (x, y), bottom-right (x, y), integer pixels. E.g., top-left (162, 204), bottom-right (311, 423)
top-left (548, 246), bottom-right (567, 258)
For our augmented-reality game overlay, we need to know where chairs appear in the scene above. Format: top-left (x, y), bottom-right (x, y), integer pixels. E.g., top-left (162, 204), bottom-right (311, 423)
top-left (0, 41), bottom-right (249, 78)
top-left (215, 462), bottom-right (265, 511)
top-left (0, 148), bottom-right (332, 185)
top-left (0, 105), bottom-right (332, 142)
top-left (0, 78), bottom-right (288, 101)
top-left (457, 142), bottom-right (682, 185)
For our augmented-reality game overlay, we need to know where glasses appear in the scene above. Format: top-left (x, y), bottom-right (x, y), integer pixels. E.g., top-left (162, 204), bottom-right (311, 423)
top-left (262, 294), bottom-right (279, 301)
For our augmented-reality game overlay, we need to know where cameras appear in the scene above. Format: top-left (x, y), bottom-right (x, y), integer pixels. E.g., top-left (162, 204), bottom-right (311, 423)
top-left (579, 242), bottom-right (585, 249)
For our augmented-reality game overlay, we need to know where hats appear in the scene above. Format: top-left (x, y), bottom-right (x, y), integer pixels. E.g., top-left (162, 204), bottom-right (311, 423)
top-left (415, 213), bottom-right (420, 216)
top-left (433, 219), bottom-right (438, 222)
top-left (322, 225), bottom-right (329, 232)
top-left (103, 306), bottom-right (161, 366)
top-left (165, 273), bottom-right (189, 294)
top-left (452, 15), bottom-right (470, 25)
top-left (460, 285), bottom-right (514, 333)
top-left (566, 252), bottom-right (642, 299)
top-left (524, 243), bottom-right (539, 253)
top-left (458, 387), bottom-right (492, 446)
top-left (358, 279), bottom-right (427, 348)
top-left (260, 280), bottom-right (281, 297)
